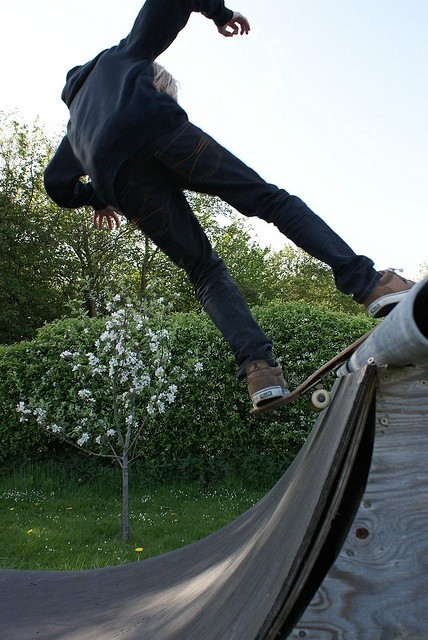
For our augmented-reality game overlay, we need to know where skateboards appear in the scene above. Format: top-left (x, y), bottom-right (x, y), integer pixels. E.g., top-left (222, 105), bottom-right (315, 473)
top-left (249, 325), bottom-right (379, 413)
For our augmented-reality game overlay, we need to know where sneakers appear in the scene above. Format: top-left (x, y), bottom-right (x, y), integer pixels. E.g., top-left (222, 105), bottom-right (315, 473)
top-left (364, 268), bottom-right (417, 319)
top-left (244, 359), bottom-right (291, 409)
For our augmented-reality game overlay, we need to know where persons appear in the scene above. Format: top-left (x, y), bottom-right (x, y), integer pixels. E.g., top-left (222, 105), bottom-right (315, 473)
top-left (43, 0), bottom-right (417, 410)
